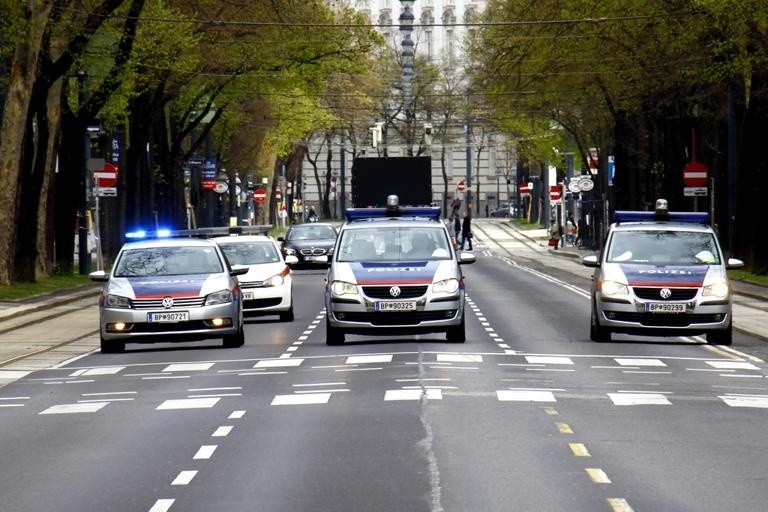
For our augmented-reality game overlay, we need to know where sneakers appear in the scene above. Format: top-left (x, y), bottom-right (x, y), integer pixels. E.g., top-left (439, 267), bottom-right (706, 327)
top-left (459, 246), bottom-right (473, 251)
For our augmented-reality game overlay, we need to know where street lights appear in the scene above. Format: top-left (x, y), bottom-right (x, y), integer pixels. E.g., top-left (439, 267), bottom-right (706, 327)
top-left (197, 95), bottom-right (219, 228)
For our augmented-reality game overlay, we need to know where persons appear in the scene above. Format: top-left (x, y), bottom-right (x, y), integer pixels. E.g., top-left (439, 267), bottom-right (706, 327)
top-left (414, 233), bottom-right (433, 254)
top-left (454, 212), bottom-right (463, 246)
top-left (547, 219), bottom-right (561, 250)
top-left (574, 213), bottom-right (590, 245)
top-left (305, 205), bottom-right (319, 223)
top-left (446, 217), bottom-right (457, 251)
top-left (565, 212), bottom-right (577, 243)
top-left (458, 211), bottom-right (474, 251)
top-left (450, 197), bottom-right (461, 218)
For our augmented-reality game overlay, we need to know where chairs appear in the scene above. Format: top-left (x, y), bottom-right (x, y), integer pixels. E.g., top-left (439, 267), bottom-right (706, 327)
top-left (245, 245), bottom-right (273, 264)
top-left (341, 239), bottom-right (371, 263)
top-left (173, 249), bottom-right (211, 275)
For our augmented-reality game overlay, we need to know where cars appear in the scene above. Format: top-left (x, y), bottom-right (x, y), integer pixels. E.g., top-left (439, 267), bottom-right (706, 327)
top-left (197, 224), bottom-right (294, 324)
top-left (312, 195), bottom-right (477, 348)
top-left (88, 228), bottom-right (245, 351)
top-left (581, 198), bottom-right (742, 345)
top-left (278, 224), bottom-right (340, 269)
top-left (491, 201), bottom-right (524, 218)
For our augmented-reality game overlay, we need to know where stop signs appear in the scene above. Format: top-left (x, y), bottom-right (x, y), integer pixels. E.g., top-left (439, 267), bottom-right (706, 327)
top-left (549, 187), bottom-right (561, 200)
top-left (254, 189), bottom-right (266, 202)
top-left (682, 162), bottom-right (708, 186)
top-left (516, 182), bottom-right (531, 195)
top-left (456, 182), bottom-right (465, 191)
top-left (92, 164), bottom-right (117, 187)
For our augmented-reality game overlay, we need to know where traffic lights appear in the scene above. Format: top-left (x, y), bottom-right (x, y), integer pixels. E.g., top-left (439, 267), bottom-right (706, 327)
top-left (368, 127), bottom-right (377, 148)
top-left (375, 122), bottom-right (383, 144)
top-left (424, 125), bottom-right (433, 145)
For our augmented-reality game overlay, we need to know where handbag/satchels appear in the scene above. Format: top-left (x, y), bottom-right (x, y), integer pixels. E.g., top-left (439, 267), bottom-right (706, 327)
top-left (467, 233), bottom-right (473, 238)
top-left (548, 238), bottom-right (557, 246)
